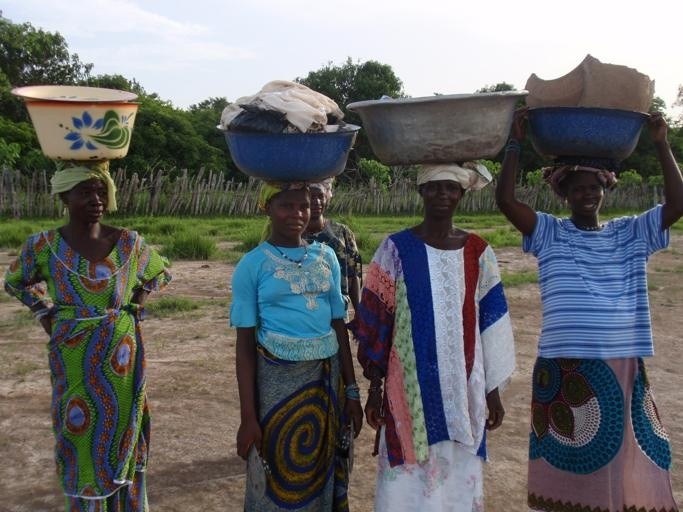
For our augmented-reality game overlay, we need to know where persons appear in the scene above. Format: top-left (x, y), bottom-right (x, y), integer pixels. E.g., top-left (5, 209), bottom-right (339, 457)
top-left (345, 162), bottom-right (517, 512)
top-left (495, 104), bottom-right (683, 512)
top-left (228, 182), bottom-right (365, 512)
top-left (3, 160), bottom-right (174, 512)
top-left (302, 176), bottom-right (363, 310)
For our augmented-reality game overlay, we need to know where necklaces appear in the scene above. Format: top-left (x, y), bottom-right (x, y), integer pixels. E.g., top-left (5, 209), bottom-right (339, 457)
top-left (271, 241), bottom-right (309, 268)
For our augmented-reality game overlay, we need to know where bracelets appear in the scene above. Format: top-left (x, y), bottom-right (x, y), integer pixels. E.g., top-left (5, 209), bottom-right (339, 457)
top-left (504, 137), bottom-right (521, 155)
top-left (345, 383), bottom-right (360, 401)
top-left (33, 308), bottom-right (50, 320)
top-left (368, 387), bottom-right (383, 395)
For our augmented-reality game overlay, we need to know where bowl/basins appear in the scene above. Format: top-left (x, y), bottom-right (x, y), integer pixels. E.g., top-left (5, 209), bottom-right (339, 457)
top-left (11, 85), bottom-right (143, 162)
top-left (344, 90), bottom-right (531, 165)
top-left (216, 123), bottom-right (361, 182)
top-left (525, 106), bottom-right (651, 158)
